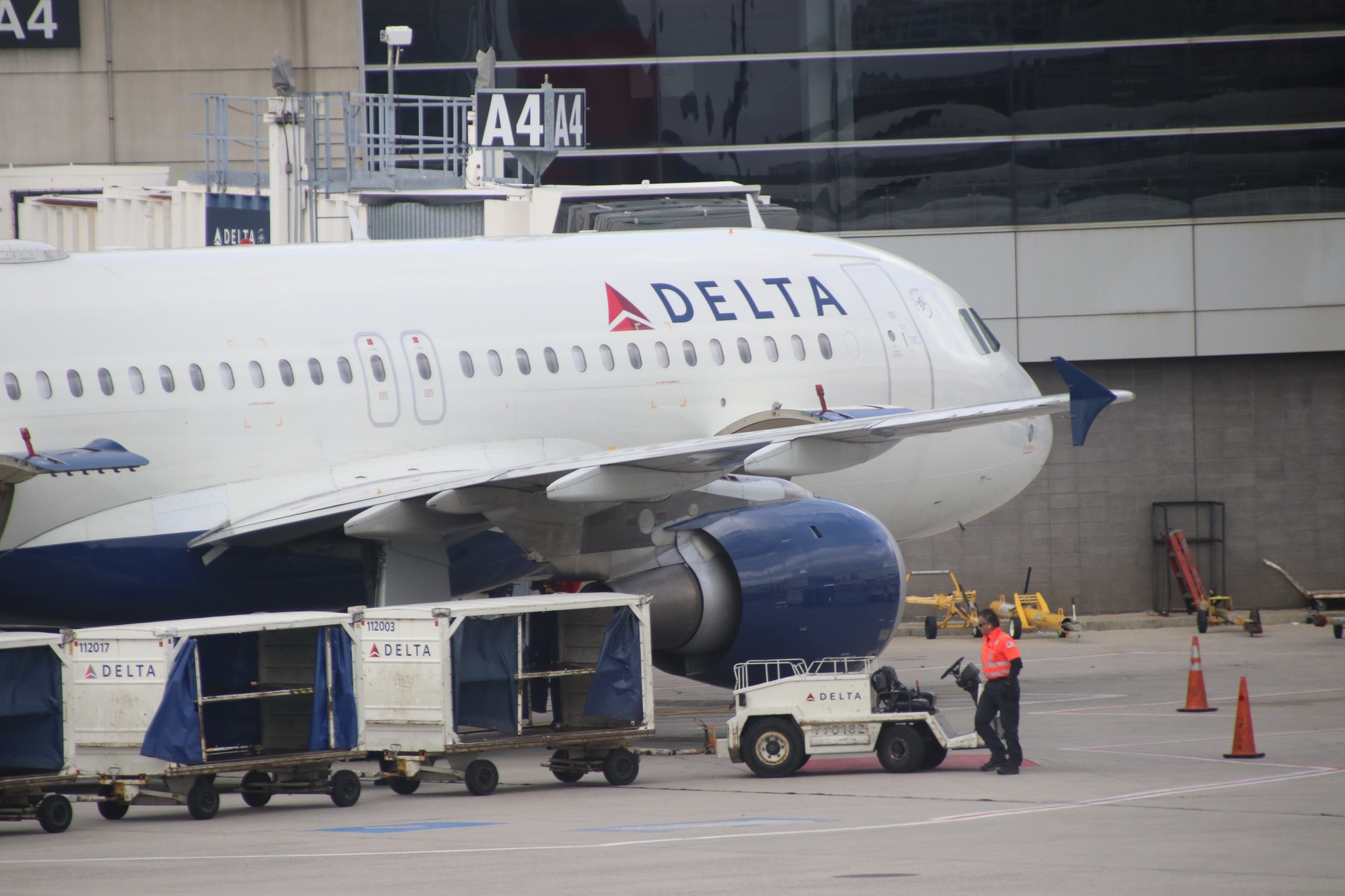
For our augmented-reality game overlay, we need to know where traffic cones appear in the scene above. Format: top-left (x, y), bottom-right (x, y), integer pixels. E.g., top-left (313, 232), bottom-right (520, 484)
top-left (1176, 636), bottom-right (1220, 713)
top-left (1222, 676), bottom-right (1266, 759)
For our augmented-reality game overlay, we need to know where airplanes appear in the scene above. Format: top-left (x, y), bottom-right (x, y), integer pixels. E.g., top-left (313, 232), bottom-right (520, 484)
top-left (0, 180), bottom-right (1136, 694)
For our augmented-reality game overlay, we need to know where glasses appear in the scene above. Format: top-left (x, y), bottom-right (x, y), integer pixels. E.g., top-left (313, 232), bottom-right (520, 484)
top-left (978, 623), bottom-right (985, 627)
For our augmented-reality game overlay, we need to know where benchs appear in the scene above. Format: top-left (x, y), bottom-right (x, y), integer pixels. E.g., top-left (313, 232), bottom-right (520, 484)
top-left (871, 666), bottom-right (937, 710)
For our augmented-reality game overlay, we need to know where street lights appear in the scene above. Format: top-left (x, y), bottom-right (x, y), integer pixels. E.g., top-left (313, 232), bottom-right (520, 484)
top-left (378, 25), bottom-right (415, 168)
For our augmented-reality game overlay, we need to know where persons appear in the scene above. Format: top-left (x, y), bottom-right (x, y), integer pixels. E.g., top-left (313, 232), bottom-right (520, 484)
top-left (976, 609), bottom-right (1023, 775)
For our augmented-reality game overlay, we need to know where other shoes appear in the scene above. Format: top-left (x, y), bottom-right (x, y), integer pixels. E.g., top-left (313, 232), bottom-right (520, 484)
top-left (997, 761), bottom-right (1020, 775)
top-left (980, 749), bottom-right (1006, 771)
